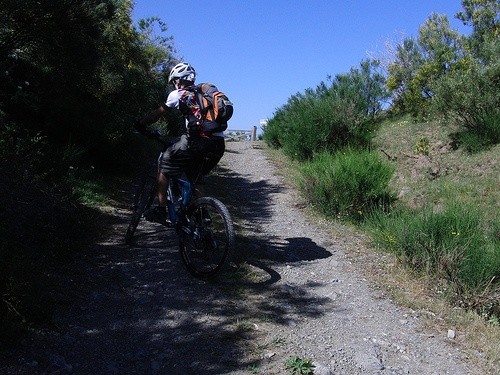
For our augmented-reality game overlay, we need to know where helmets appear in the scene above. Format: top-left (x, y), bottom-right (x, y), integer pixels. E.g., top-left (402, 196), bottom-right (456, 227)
top-left (168, 62), bottom-right (196, 83)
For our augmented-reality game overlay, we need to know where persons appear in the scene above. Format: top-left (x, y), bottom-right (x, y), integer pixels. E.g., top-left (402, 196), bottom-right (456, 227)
top-left (137, 62), bottom-right (226, 228)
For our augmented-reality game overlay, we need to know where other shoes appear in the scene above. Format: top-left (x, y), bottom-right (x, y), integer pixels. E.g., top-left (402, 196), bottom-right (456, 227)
top-left (194, 209), bottom-right (212, 226)
top-left (144, 206), bottom-right (167, 224)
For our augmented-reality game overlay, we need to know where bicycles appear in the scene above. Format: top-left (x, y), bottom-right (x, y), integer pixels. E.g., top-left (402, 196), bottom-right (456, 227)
top-left (124, 116), bottom-right (235, 280)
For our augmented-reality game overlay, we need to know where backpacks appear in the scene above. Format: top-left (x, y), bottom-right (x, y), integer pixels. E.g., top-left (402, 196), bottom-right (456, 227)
top-left (179, 83), bottom-right (233, 140)
